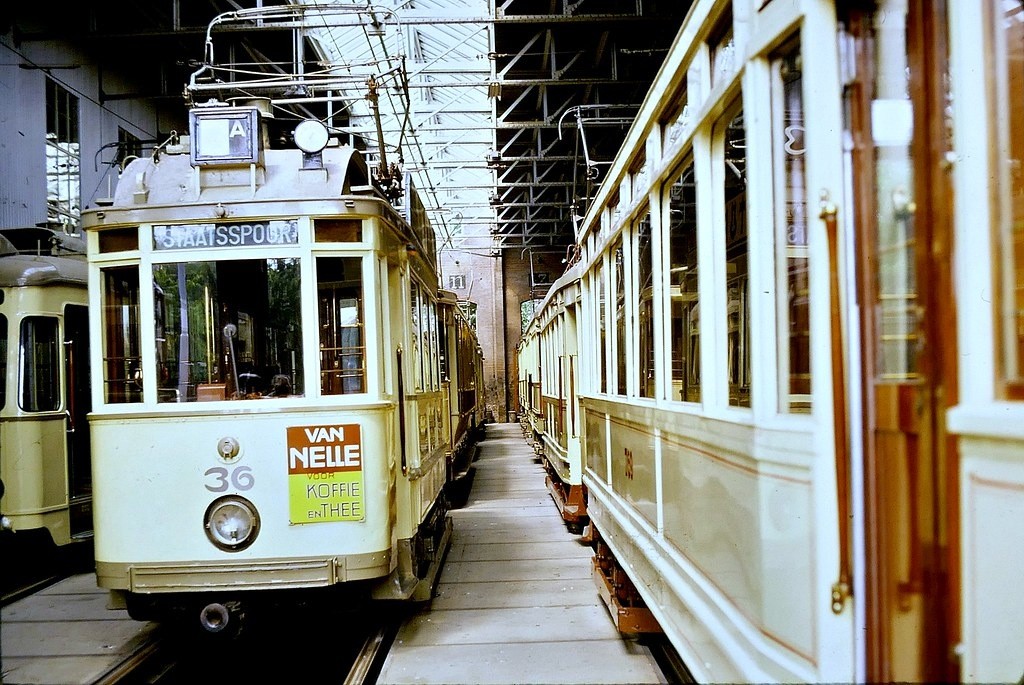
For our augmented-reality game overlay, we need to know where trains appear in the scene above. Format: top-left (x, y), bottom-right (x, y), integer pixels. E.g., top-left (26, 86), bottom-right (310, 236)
top-left (0, 222), bottom-right (252, 551)
top-left (80, 95), bottom-right (487, 636)
top-left (519, 1), bottom-right (1023, 685)
